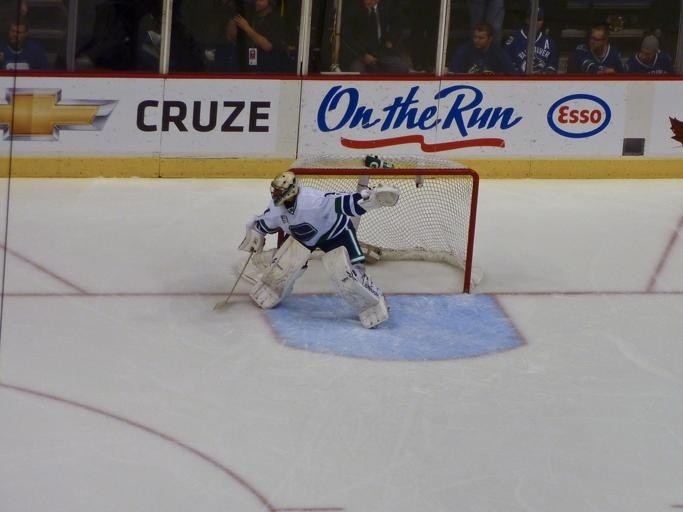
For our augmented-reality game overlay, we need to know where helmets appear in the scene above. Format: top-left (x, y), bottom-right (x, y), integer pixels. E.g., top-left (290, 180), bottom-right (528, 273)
top-left (270, 171), bottom-right (299, 206)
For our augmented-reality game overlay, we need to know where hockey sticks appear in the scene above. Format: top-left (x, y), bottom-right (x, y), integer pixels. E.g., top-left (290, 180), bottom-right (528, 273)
top-left (212, 251), bottom-right (254, 310)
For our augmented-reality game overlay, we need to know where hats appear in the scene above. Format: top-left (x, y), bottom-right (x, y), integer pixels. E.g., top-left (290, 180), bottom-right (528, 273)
top-left (641, 35), bottom-right (659, 54)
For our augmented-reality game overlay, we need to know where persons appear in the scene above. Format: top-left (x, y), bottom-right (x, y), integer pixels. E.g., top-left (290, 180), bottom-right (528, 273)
top-left (237, 170), bottom-right (401, 331)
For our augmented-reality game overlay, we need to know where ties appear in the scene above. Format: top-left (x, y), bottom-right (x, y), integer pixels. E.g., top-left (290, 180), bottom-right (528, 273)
top-left (369, 8), bottom-right (381, 66)
